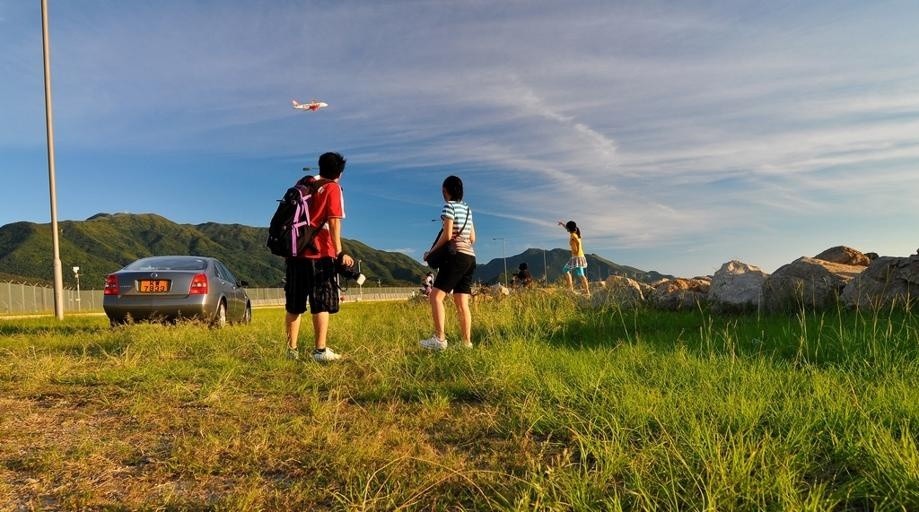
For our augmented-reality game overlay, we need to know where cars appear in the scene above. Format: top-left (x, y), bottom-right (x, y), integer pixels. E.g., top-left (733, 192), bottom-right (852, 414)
top-left (103, 255), bottom-right (252, 330)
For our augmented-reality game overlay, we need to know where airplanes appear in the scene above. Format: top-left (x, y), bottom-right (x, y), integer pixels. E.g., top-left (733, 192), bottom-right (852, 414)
top-left (290, 99), bottom-right (329, 113)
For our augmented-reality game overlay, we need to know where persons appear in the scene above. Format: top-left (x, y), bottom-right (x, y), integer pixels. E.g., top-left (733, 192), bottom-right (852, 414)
top-left (418, 174), bottom-right (479, 349)
top-left (419, 270), bottom-right (434, 297)
top-left (558, 220), bottom-right (591, 295)
top-left (516, 262), bottom-right (533, 288)
top-left (281, 149), bottom-right (354, 360)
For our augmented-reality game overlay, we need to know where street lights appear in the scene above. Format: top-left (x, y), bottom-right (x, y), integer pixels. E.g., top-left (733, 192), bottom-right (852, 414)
top-left (492, 237), bottom-right (508, 288)
top-left (431, 218), bottom-right (441, 222)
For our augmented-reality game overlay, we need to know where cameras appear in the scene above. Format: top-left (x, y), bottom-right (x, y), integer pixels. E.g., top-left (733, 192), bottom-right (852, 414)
top-left (328, 255), bottom-right (366, 286)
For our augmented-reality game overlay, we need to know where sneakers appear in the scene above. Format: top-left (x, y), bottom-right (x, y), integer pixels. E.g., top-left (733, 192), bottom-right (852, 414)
top-left (419, 336), bottom-right (447, 351)
top-left (314, 348), bottom-right (340, 361)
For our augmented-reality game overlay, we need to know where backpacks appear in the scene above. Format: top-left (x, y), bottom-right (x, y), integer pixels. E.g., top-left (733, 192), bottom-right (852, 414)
top-left (266, 176), bottom-right (336, 257)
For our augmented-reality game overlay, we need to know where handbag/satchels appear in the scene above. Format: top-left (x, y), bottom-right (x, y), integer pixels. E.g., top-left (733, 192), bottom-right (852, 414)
top-left (428, 241), bottom-right (458, 268)
